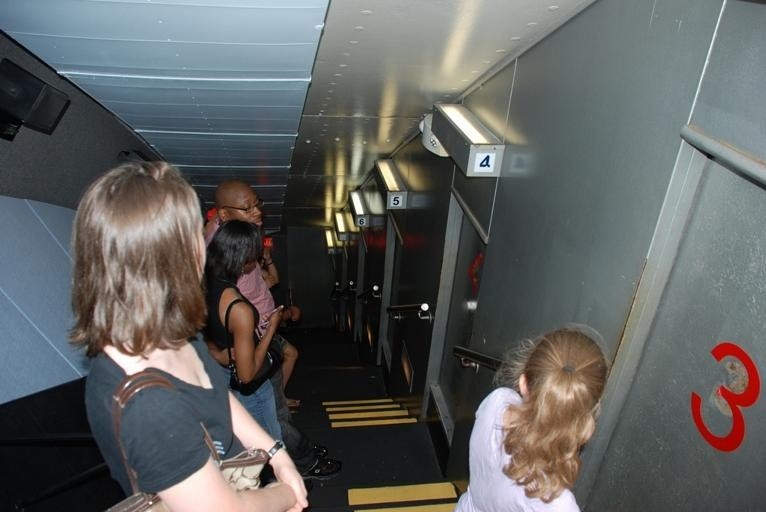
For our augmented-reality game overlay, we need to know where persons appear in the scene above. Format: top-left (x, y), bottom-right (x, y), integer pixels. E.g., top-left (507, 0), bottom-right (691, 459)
top-left (454, 328), bottom-right (606, 511)
top-left (63, 159), bottom-right (310, 512)
top-left (200, 179), bottom-right (342, 480)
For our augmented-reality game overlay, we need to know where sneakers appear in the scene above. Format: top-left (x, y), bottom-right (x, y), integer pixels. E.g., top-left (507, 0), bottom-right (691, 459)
top-left (285, 399), bottom-right (301, 412)
top-left (301, 457), bottom-right (343, 481)
top-left (314, 443), bottom-right (329, 457)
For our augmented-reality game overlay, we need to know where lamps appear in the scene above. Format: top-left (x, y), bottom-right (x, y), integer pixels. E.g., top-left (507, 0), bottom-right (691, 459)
top-left (322, 103), bottom-right (506, 254)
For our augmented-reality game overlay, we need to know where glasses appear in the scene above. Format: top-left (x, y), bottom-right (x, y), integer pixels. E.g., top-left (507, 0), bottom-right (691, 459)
top-left (222, 200), bottom-right (265, 213)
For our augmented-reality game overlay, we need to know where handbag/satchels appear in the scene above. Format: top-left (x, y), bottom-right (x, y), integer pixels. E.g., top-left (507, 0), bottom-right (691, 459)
top-left (224, 299), bottom-right (284, 397)
top-left (104, 371), bottom-right (270, 512)
